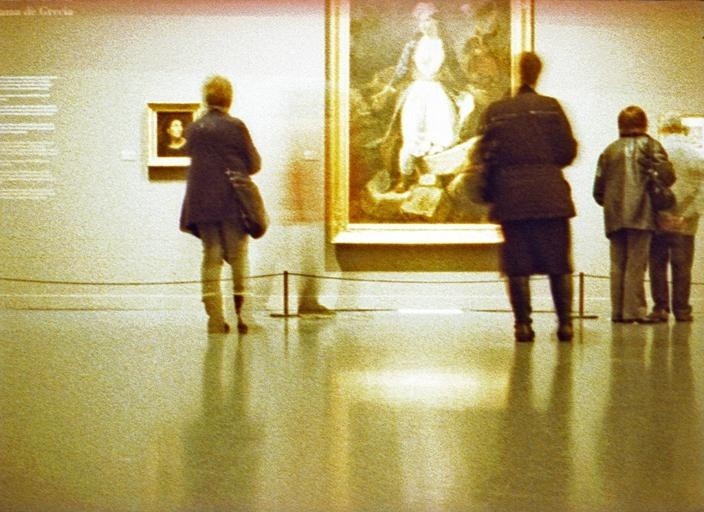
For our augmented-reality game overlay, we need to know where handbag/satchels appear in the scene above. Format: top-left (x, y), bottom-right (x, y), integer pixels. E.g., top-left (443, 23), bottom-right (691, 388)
top-left (223, 169), bottom-right (270, 238)
top-left (467, 132), bottom-right (494, 205)
top-left (646, 167), bottom-right (677, 211)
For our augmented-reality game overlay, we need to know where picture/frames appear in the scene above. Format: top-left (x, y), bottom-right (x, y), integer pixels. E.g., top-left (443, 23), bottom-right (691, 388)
top-left (147, 102), bottom-right (202, 169)
top-left (322, 0), bottom-right (533, 249)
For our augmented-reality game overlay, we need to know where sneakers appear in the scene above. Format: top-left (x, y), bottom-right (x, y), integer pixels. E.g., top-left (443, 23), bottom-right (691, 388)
top-left (514, 325), bottom-right (535, 341)
top-left (208, 320), bottom-right (230, 335)
top-left (557, 326), bottom-right (574, 341)
top-left (611, 304), bottom-right (696, 324)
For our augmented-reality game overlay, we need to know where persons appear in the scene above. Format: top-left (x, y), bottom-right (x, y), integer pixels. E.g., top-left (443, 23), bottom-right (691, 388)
top-left (470, 49), bottom-right (579, 339)
top-left (648, 110), bottom-right (703, 322)
top-left (179, 74), bottom-right (262, 334)
top-left (369, 6), bottom-right (475, 191)
top-left (592, 104), bottom-right (676, 325)
top-left (159, 117), bottom-right (191, 156)
